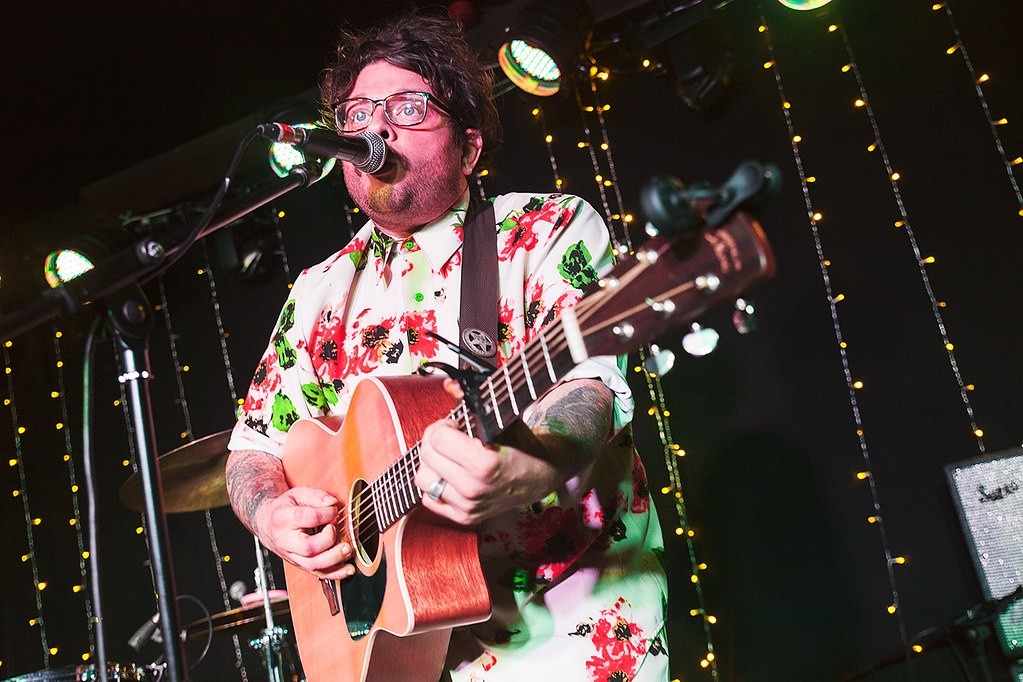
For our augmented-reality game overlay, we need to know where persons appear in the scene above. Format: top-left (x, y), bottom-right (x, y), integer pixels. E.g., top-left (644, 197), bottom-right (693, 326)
top-left (223, 14), bottom-right (677, 682)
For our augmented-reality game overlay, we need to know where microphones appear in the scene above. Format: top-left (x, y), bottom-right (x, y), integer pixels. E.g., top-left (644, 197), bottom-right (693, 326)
top-left (258, 122), bottom-right (393, 174)
top-left (126, 612), bottom-right (161, 653)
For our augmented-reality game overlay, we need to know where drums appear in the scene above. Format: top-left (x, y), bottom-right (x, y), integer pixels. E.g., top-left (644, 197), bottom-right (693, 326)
top-left (4, 662), bottom-right (149, 682)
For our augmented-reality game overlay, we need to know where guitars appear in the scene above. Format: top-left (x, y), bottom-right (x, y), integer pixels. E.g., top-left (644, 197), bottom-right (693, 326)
top-left (281, 182), bottom-right (777, 682)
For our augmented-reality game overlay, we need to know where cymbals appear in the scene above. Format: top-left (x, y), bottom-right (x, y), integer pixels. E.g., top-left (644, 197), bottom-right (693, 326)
top-left (188, 597), bottom-right (291, 638)
top-left (118, 428), bottom-right (235, 513)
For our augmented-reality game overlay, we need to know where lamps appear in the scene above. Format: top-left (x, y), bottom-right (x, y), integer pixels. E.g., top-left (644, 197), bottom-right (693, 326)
top-left (498, 0), bottom-right (596, 97)
top-left (29, 225), bottom-right (125, 306)
top-left (264, 97), bottom-right (337, 187)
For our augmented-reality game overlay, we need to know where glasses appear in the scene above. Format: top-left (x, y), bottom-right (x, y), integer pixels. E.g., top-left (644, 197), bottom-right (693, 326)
top-left (330, 91), bottom-right (461, 133)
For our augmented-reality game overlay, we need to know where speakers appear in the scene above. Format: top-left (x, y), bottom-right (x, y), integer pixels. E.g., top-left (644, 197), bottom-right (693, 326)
top-left (943, 443), bottom-right (1023, 660)
top-left (1006, 659), bottom-right (1023, 682)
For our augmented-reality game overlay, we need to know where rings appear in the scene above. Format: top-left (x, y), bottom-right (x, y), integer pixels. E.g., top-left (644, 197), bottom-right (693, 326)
top-left (428, 478), bottom-right (448, 502)
